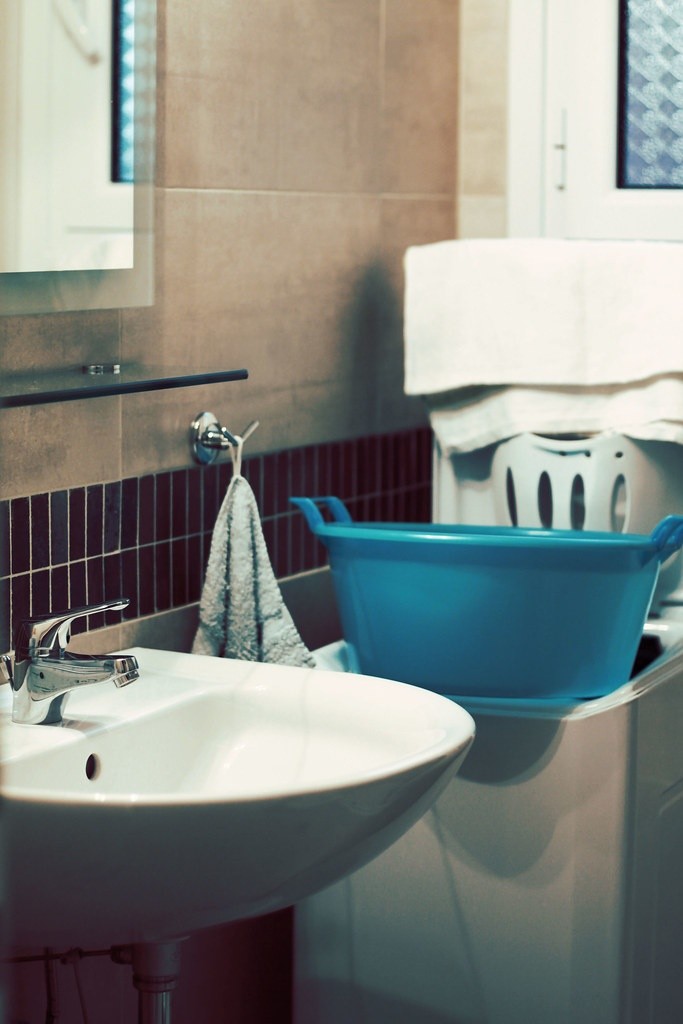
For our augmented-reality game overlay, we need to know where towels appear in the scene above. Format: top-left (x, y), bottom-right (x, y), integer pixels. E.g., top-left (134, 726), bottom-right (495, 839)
top-left (187, 475), bottom-right (319, 670)
top-left (401, 234), bottom-right (682, 459)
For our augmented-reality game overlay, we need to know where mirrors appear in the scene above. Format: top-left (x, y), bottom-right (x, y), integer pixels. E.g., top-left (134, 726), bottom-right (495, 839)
top-left (1, 0), bottom-right (159, 310)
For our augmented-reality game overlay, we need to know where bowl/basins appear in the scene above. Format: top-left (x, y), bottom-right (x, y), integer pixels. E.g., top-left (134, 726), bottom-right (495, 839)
top-left (292, 494), bottom-right (683, 699)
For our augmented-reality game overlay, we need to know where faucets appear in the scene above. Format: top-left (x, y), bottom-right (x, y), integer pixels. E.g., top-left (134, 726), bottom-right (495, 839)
top-left (10, 595), bottom-right (140, 725)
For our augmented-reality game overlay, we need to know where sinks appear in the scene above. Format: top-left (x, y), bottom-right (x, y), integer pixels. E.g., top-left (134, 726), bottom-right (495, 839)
top-left (0, 646), bottom-right (479, 967)
